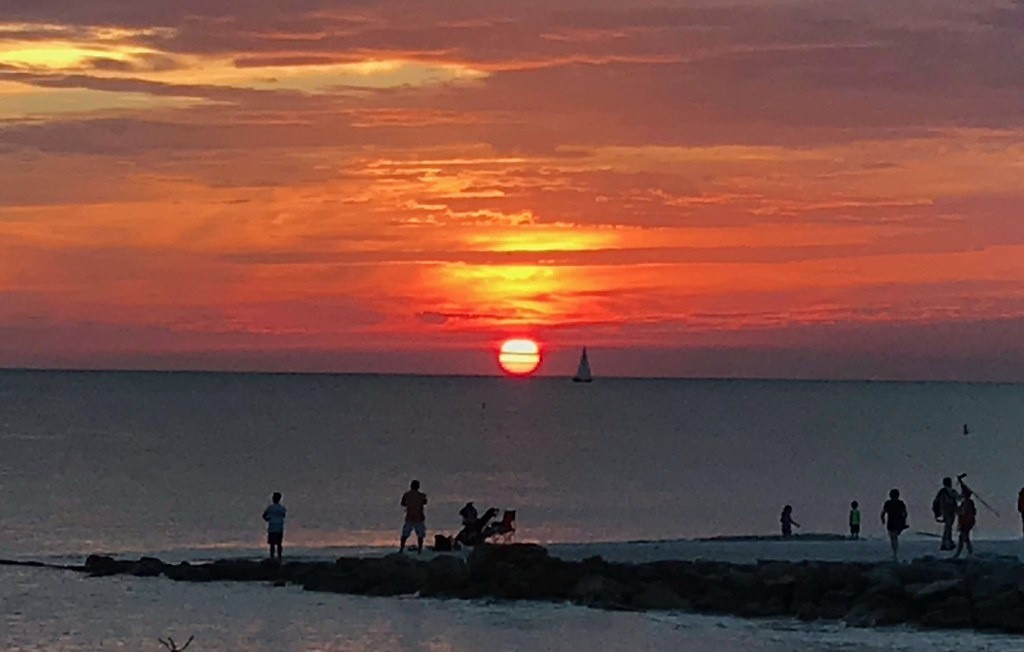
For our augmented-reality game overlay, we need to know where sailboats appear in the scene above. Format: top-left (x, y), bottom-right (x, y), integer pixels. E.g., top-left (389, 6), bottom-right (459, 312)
top-left (572, 345), bottom-right (592, 383)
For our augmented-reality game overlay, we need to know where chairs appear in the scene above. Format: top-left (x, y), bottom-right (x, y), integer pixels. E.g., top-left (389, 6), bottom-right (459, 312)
top-left (491, 510), bottom-right (516, 545)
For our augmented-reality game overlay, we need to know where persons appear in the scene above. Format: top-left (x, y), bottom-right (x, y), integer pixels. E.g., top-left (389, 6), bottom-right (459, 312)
top-left (952, 490), bottom-right (978, 560)
top-left (848, 500), bottom-right (861, 541)
top-left (458, 502), bottom-right (478, 527)
top-left (779, 505), bottom-right (800, 537)
top-left (262, 491), bottom-right (287, 558)
top-left (932, 476), bottom-right (958, 551)
top-left (878, 489), bottom-right (908, 560)
top-left (1017, 487), bottom-right (1024, 535)
top-left (397, 480), bottom-right (428, 555)
